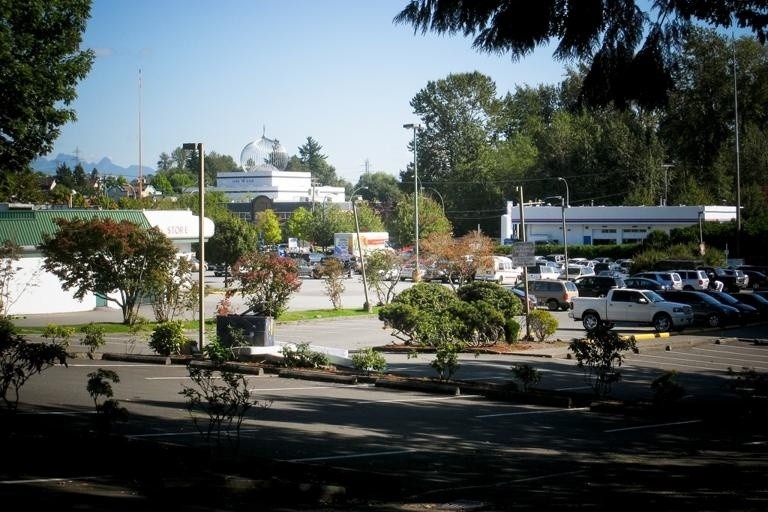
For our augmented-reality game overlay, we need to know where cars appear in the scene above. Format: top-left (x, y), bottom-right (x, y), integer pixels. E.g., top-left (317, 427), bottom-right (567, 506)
top-left (207, 264), bottom-right (249, 276)
top-left (558, 265), bottom-right (595, 281)
top-left (507, 289), bottom-right (537, 314)
top-left (707, 291), bottom-right (760, 325)
top-left (512, 253), bottom-right (636, 278)
top-left (622, 278), bottom-right (666, 291)
top-left (652, 289), bottom-right (742, 328)
top-left (280, 249), bottom-right (475, 286)
top-left (694, 265), bottom-right (766, 291)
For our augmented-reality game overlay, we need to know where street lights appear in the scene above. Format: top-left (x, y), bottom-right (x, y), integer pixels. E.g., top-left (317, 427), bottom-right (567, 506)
top-left (348, 187), bottom-right (369, 209)
top-left (403, 123), bottom-right (422, 283)
top-left (557, 177), bottom-right (569, 207)
top-left (352, 194), bottom-right (373, 313)
top-left (183, 142), bottom-right (206, 353)
top-left (410, 174), bottom-right (445, 219)
top-left (323, 192), bottom-right (338, 254)
top-left (697, 210), bottom-right (706, 254)
top-left (545, 195), bottom-right (570, 279)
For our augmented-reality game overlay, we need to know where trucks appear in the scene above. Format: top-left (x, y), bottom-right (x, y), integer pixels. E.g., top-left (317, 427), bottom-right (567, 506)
top-left (333, 232), bottom-right (396, 267)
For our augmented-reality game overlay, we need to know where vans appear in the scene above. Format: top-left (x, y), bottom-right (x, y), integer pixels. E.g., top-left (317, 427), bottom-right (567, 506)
top-left (475, 256), bottom-right (522, 287)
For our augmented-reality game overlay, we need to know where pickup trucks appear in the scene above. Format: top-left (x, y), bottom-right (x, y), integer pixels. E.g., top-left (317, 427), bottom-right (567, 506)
top-left (567, 287), bottom-right (694, 332)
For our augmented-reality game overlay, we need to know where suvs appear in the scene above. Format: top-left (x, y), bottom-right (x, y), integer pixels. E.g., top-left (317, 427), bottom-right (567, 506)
top-left (511, 279), bottom-right (580, 311)
top-left (650, 259), bottom-right (705, 272)
top-left (571, 276), bottom-right (627, 298)
top-left (631, 271), bottom-right (682, 290)
top-left (522, 264), bottom-right (560, 280)
top-left (667, 270), bottom-right (711, 291)
